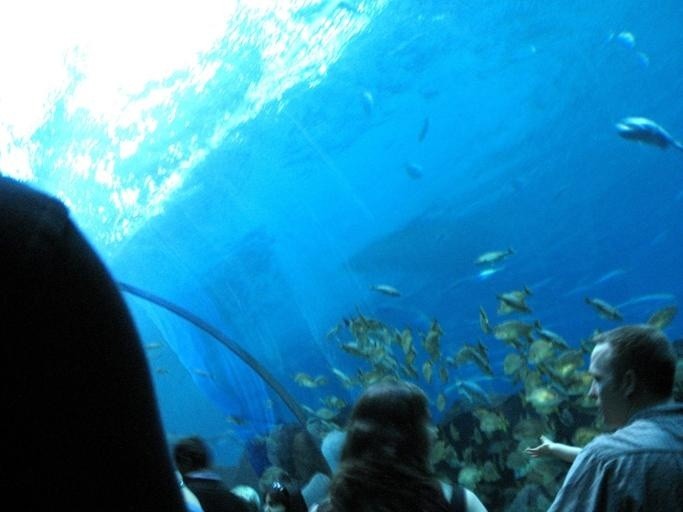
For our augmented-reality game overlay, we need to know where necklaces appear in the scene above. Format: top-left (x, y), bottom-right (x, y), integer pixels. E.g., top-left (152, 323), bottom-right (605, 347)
top-left (167, 433), bottom-right (257, 512)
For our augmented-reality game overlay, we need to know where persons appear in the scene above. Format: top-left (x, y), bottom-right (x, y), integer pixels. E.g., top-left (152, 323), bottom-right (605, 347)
top-left (260, 477), bottom-right (309, 511)
top-left (0, 164), bottom-right (191, 510)
top-left (522, 434), bottom-right (585, 464)
top-left (227, 483), bottom-right (261, 511)
top-left (319, 378), bottom-right (490, 511)
top-left (543, 320), bottom-right (683, 511)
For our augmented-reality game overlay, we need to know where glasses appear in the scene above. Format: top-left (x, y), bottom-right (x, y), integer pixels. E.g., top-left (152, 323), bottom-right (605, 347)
top-left (271, 481), bottom-right (289, 505)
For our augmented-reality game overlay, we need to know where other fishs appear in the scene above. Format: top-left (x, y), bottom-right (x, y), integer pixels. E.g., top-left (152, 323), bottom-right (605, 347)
top-left (292, 284), bottom-right (682, 511)
